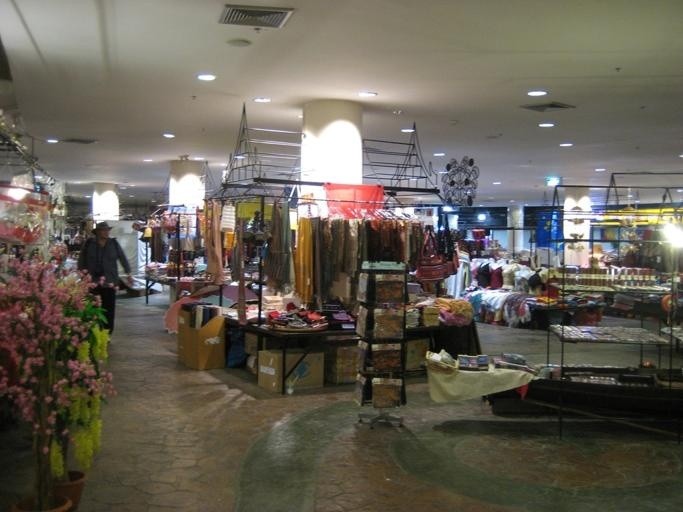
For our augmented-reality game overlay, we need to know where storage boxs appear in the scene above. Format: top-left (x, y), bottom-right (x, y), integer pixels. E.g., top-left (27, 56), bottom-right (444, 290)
top-left (177, 307), bottom-right (324, 395)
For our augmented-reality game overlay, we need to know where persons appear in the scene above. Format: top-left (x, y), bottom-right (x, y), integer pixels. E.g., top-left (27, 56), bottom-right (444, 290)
top-left (73, 221), bottom-right (134, 343)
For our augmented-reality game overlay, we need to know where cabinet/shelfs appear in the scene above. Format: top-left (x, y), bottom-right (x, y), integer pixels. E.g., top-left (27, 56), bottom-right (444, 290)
top-left (545, 185), bottom-right (683, 440)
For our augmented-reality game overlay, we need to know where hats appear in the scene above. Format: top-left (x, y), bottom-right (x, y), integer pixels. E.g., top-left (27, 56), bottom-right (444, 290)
top-left (92, 221), bottom-right (112, 235)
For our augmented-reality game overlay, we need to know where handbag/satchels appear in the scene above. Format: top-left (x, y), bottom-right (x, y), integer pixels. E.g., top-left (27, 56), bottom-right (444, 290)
top-left (417, 229), bottom-right (459, 283)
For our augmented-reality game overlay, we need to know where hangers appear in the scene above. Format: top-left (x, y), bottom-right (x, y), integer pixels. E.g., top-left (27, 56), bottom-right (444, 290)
top-left (296, 197), bottom-right (412, 220)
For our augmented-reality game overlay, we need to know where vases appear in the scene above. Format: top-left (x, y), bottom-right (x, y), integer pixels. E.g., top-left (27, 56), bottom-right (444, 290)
top-left (7, 471), bottom-right (87, 511)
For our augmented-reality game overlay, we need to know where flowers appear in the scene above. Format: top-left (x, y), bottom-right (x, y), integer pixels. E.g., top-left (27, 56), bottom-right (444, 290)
top-left (0, 247), bottom-right (118, 509)
